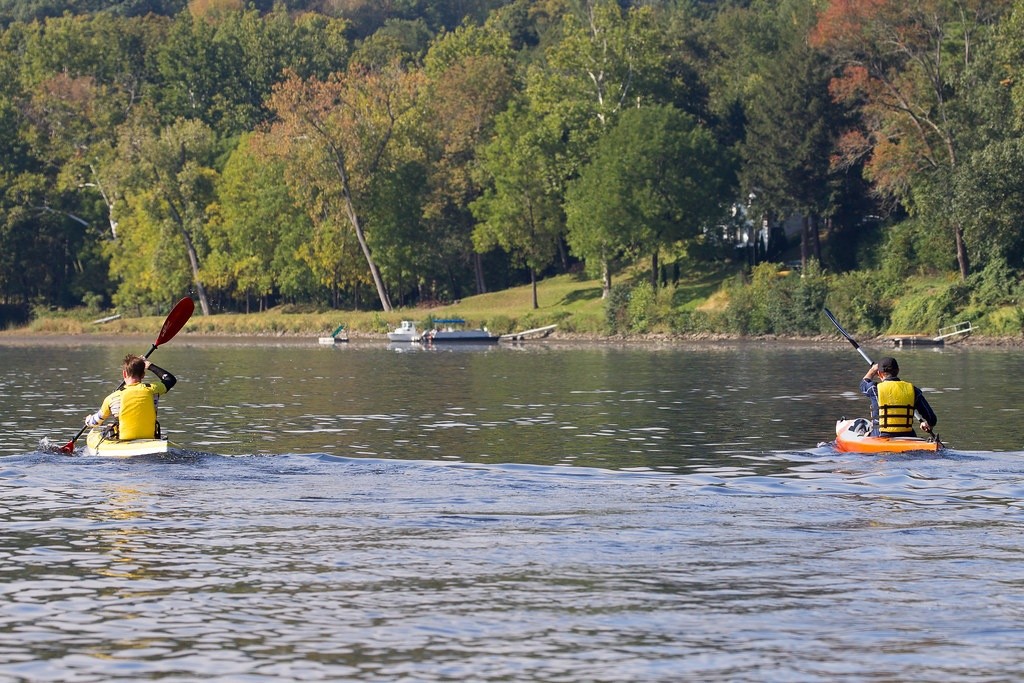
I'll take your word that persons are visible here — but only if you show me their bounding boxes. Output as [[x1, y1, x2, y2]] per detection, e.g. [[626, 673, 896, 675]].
[[859, 357, 937, 438], [85, 354, 177, 440]]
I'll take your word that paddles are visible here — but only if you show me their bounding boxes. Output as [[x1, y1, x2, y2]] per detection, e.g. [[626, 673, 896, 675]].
[[822, 307, 946, 448], [59, 296, 197, 456]]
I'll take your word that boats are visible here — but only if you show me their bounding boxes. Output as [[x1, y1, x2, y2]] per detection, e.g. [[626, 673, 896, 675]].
[[387, 320, 422, 342], [317, 325, 349, 344], [835, 415, 940, 455], [411, 317, 501, 343], [85, 424, 169, 458]]
